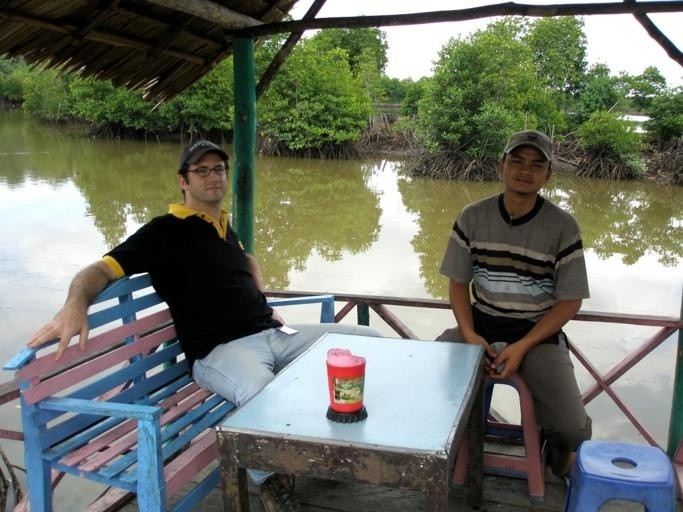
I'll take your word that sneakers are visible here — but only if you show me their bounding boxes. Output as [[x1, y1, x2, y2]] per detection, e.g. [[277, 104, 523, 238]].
[[550, 445, 571, 475], [258, 477, 302, 512]]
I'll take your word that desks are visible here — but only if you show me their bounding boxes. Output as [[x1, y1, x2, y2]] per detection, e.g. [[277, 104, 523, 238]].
[[215, 331, 485, 512]]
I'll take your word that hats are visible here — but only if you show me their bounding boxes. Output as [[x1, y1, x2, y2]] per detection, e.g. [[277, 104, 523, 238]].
[[178, 141, 229, 173], [503, 130, 553, 162]]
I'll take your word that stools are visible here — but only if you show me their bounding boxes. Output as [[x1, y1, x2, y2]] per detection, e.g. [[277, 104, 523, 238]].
[[452, 372, 547, 503], [563, 440, 676, 512]]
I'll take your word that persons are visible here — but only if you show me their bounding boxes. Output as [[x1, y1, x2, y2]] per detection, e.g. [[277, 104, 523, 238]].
[[433, 128, 593, 477], [24, 137, 385, 512]]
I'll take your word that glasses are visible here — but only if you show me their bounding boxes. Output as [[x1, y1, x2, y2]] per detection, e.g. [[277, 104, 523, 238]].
[[188, 166, 228, 177]]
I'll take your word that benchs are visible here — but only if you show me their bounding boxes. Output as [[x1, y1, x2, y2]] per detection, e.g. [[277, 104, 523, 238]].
[[3, 274, 335, 512]]
